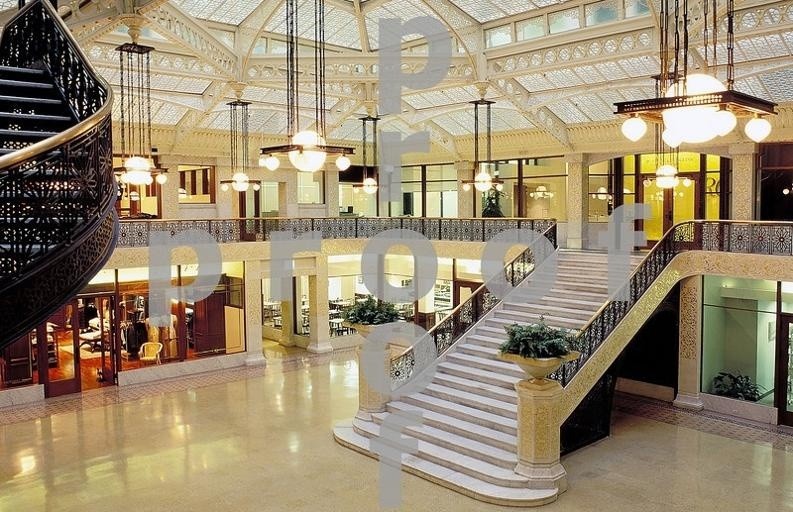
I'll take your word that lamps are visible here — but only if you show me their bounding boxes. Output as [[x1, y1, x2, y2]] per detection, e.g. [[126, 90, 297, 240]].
[[107, 0, 505, 195], [611, 4, 779, 191]]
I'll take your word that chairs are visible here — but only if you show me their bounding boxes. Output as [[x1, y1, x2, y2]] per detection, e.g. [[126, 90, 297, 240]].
[[137, 342, 163, 366]]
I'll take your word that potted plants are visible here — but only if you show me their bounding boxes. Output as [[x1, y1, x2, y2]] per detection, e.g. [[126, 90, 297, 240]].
[[493, 311, 584, 382], [340, 294, 406, 345]]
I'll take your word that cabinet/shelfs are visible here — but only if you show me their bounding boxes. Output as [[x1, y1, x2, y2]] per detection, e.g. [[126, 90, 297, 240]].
[[30, 331, 58, 368]]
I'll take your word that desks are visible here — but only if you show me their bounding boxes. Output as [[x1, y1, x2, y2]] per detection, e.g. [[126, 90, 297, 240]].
[[301, 301, 356, 336], [78, 332, 101, 352], [263, 302, 281, 331]]
